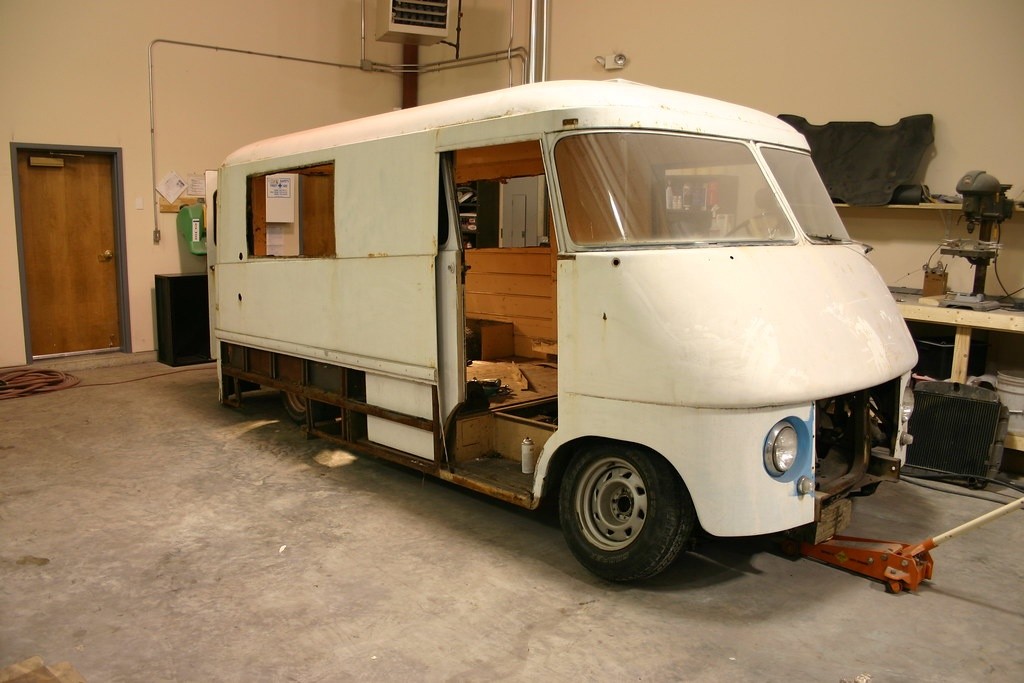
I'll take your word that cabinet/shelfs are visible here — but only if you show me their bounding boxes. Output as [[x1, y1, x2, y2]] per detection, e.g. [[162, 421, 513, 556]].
[[832, 197, 1023, 454], [156, 273, 217, 367]]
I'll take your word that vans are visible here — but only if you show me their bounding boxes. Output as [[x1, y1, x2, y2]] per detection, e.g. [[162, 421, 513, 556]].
[[207, 78, 918, 582]]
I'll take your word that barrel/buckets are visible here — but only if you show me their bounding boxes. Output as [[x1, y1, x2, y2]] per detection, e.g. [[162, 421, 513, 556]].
[[995, 369, 1024, 436]]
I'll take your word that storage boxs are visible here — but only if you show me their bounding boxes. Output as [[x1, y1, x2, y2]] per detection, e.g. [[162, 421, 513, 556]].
[[465, 319, 515, 362]]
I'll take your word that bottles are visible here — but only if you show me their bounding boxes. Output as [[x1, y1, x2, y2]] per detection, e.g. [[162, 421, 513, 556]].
[[521, 436, 535, 474], [666, 183, 691, 210]]
